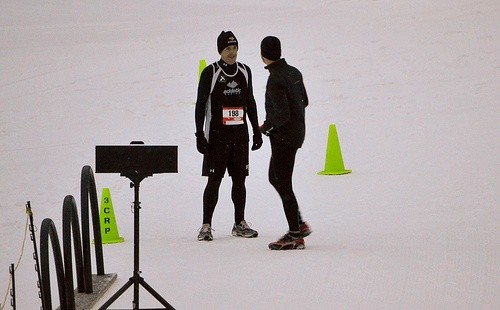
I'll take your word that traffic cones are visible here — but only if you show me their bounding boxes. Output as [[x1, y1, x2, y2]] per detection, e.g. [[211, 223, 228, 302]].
[[90, 187, 126, 244], [197, 59, 208, 80], [316, 123, 352, 176]]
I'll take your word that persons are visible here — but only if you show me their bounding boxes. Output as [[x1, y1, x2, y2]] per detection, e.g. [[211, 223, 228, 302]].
[[194, 30, 263, 241], [259, 36, 313, 250]]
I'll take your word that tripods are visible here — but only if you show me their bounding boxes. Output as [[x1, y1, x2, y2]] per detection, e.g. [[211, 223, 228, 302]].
[[93, 140, 188, 310]]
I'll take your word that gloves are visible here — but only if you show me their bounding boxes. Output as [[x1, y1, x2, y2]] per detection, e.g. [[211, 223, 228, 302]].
[[195, 131, 208, 154], [259, 121, 274, 136], [251, 133, 263, 150]]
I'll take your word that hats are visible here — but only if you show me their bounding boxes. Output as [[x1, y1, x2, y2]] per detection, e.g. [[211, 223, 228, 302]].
[[217, 30, 238, 54], [261, 36, 281, 61]]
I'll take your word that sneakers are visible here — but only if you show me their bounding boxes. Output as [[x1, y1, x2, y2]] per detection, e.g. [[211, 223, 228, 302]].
[[299, 222, 312, 237], [198, 223, 215, 241], [268, 232, 305, 250], [232, 220, 258, 238]]
